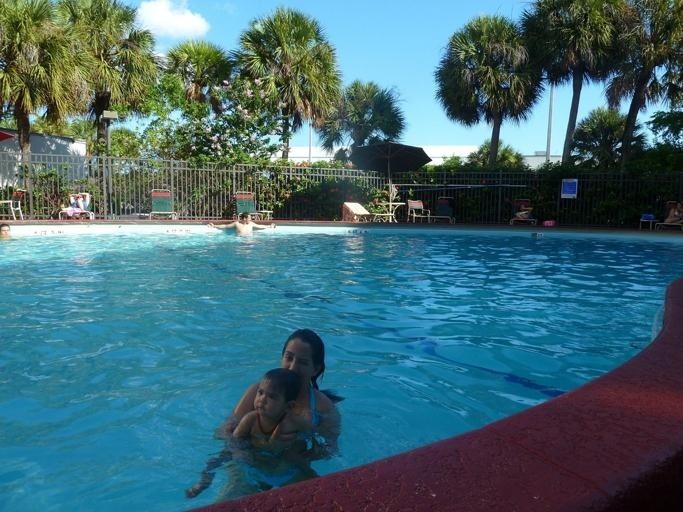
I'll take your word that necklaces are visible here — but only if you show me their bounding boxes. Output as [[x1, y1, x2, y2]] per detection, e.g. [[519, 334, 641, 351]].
[[256, 414, 285, 436]]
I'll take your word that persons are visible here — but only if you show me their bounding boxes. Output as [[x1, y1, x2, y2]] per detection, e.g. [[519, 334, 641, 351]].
[[512, 204, 533, 219], [214, 328, 339, 505], [0, 223, 10, 236], [185, 367, 310, 498], [68, 192, 90, 211], [664, 202, 682, 223], [207, 213, 275, 237]]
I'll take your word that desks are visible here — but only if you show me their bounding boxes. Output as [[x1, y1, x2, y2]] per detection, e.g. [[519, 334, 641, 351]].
[[639, 219, 660, 231]]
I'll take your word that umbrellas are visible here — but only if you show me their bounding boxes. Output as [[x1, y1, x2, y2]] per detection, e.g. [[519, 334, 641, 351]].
[[348, 140, 433, 213]]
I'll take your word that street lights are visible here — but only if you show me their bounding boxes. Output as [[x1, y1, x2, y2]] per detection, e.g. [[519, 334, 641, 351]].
[[99, 110, 118, 218]]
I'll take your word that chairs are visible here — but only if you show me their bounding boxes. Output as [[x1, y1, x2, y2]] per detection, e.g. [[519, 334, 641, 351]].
[[508, 198, 539, 227], [58, 194, 96, 222], [234, 192, 264, 220], [343, 197, 455, 224], [150, 190, 177, 220], [1, 188, 26, 221], [654, 200, 683, 234]]
